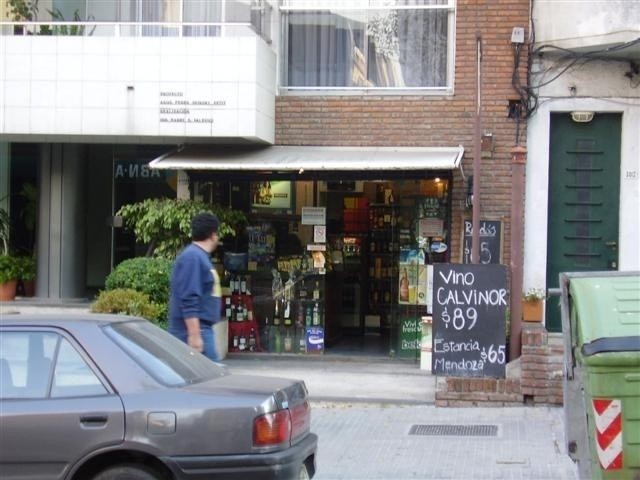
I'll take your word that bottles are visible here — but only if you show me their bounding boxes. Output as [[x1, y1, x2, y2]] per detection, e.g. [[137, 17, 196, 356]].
[[265, 275, 323, 353], [227, 273, 248, 294], [222, 297, 252, 323], [342, 244, 363, 265], [367, 207, 398, 255], [420, 195, 440, 218], [231, 327, 256, 351], [276, 256, 311, 277], [400, 268, 409, 301]]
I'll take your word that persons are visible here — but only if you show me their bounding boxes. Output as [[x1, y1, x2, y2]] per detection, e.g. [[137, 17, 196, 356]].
[[167, 213, 222, 364]]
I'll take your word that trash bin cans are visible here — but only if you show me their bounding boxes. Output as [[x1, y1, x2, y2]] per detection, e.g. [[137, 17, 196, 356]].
[[559, 271, 640, 480]]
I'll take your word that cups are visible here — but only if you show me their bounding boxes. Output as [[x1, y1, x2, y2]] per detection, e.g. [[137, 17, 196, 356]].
[[408, 285, 417, 304]]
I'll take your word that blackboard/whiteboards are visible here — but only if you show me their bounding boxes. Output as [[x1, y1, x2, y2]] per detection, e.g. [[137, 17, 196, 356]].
[[432, 263, 507, 377]]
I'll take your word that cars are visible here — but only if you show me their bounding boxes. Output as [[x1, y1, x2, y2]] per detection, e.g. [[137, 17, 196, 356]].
[[0, 313, 319, 480]]
